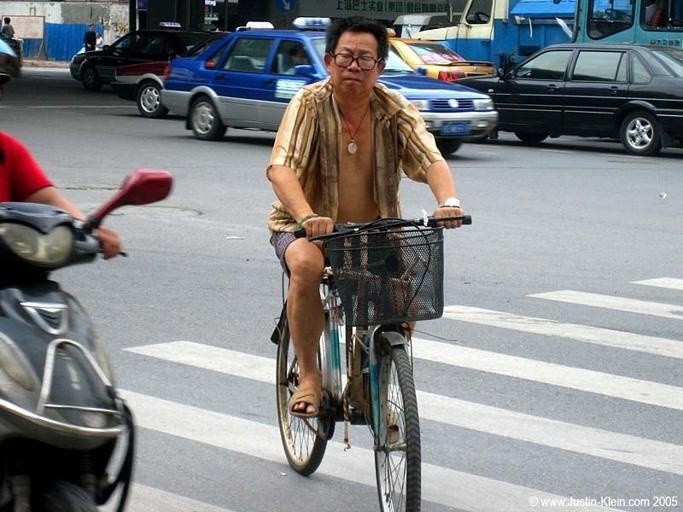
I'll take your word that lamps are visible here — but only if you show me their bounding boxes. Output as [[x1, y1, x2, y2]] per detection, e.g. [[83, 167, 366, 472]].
[[276, 54, 290, 73], [229, 56, 256, 69]]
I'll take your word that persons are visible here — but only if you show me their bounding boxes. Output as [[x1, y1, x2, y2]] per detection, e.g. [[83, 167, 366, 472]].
[[84, 24, 96, 52], [284, 42, 310, 75], [95, 32, 102, 49], [209, 19, 220, 31], [0, 17, 15, 40], [1, 129, 122, 261], [262, 18, 469, 446], [624, 0, 658, 25]]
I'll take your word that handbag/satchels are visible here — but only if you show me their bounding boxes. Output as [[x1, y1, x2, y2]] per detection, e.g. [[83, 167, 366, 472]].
[[438, 197, 461, 208], [297, 213, 319, 230]]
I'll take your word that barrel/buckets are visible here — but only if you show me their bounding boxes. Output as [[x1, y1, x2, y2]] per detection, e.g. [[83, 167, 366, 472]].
[[369, 402, 400, 445], [288, 391, 323, 418]]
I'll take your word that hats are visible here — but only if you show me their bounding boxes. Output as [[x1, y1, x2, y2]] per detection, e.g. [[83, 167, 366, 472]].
[[390, 27, 495, 82], [158, 18, 500, 153], [68, 21, 180, 93], [0, 35, 397, 106], [450, 43, 681, 154], [110, 31, 230, 117]]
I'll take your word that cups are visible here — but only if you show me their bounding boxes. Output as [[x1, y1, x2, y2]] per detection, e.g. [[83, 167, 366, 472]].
[[329, 49, 383, 72]]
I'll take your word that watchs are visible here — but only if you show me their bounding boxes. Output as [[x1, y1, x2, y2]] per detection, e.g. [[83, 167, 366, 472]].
[[1, 170, 172, 507]]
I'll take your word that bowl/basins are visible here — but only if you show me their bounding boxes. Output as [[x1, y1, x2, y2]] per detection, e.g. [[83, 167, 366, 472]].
[[338, 104, 370, 155]]
